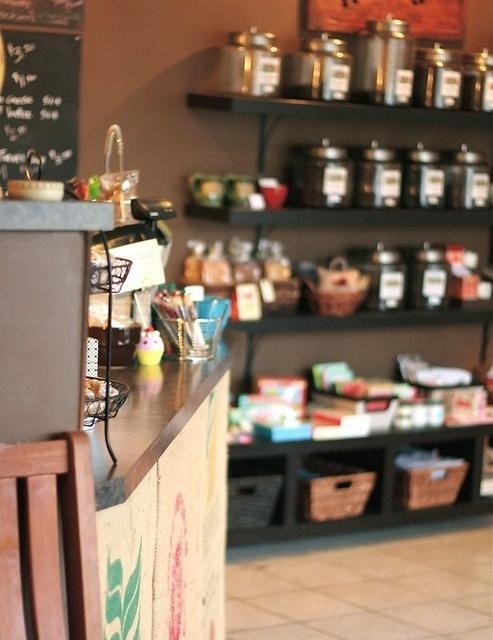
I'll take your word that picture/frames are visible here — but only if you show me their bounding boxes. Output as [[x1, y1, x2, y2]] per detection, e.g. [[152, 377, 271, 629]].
[[306, 0, 468, 42]]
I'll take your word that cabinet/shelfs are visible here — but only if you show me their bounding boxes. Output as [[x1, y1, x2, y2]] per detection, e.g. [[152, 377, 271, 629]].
[[185, 93, 493, 547]]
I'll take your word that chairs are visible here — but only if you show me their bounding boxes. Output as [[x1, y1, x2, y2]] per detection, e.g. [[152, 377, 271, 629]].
[[0, 430, 107, 640]]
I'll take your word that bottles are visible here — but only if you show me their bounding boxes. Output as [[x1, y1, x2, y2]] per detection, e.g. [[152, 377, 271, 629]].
[[298, 138, 352, 207], [401, 140, 446, 212], [442, 142, 490, 211], [413, 41, 463, 110], [219, 26, 279, 100], [287, 32, 354, 103], [182, 233, 291, 293], [404, 241, 451, 312], [355, 13, 416, 107], [463, 48, 492, 113], [354, 140, 402, 210], [357, 238, 406, 312]]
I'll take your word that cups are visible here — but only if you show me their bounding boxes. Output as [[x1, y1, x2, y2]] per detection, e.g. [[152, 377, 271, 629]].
[[258, 183, 289, 211], [186, 171, 223, 209], [222, 175, 256, 212]]
